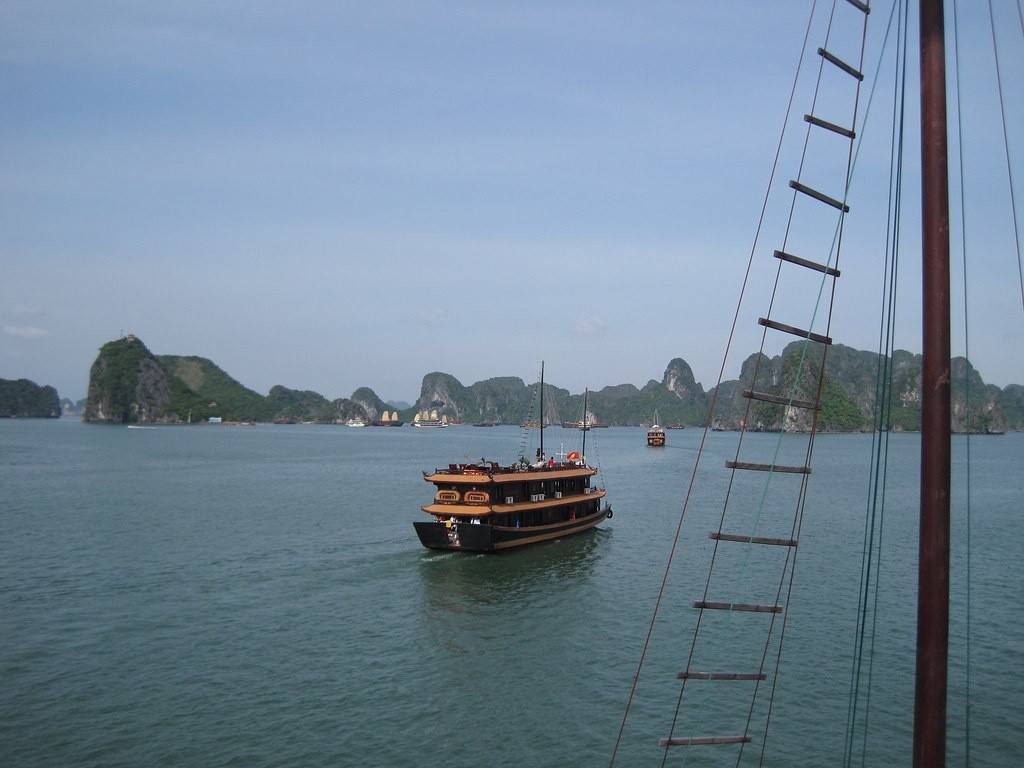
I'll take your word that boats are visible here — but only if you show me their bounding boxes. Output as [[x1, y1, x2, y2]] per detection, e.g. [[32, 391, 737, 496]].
[[413, 361, 613, 556], [578, 419, 591, 431], [348, 421, 365, 427], [647, 409, 666, 448]]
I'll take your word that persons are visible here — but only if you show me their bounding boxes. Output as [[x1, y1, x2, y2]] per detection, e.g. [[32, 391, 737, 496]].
[[548, 457, 554, 468], [517, 520, 520, 528]]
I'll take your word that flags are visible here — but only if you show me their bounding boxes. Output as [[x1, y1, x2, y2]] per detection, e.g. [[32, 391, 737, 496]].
[[567, 451, 580, 459]]
